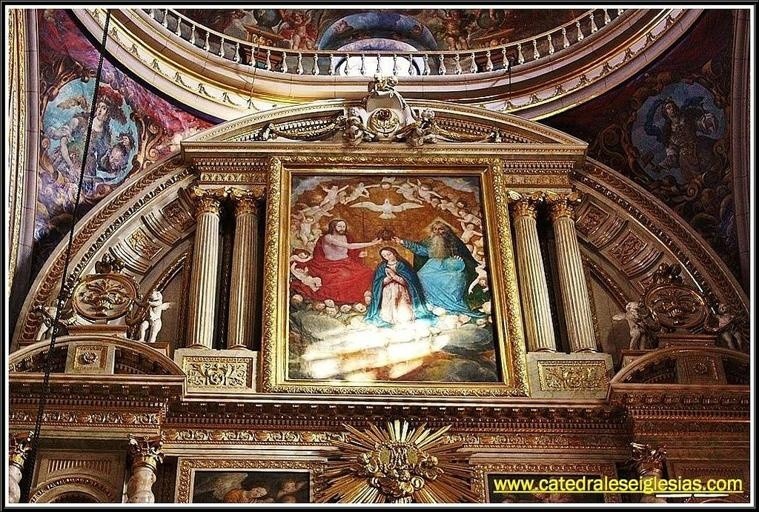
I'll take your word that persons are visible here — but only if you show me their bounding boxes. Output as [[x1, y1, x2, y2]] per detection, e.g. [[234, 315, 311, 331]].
[[50, 83, 127, 194], [279, 9, 318, 52], [220, 482, 269, 503], [289, 174, 492, 330], [499, 479, 575, 502], [135, 288, 175, 343], [116, 127, 136, 152], [611, 300, 651, 351], [653, 93, 719, 184], [437, 6, 471, 52], [278, 495, 296, 503], [274, 476, 310, 501], [32, 298, 65, 342], [712, 302, 743, 352]]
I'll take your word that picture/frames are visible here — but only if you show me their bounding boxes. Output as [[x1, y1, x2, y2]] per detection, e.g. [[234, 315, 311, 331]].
[[173, 457, 327, 503], [260, 155, 531, 398], [470, 463, 622, 504]]
